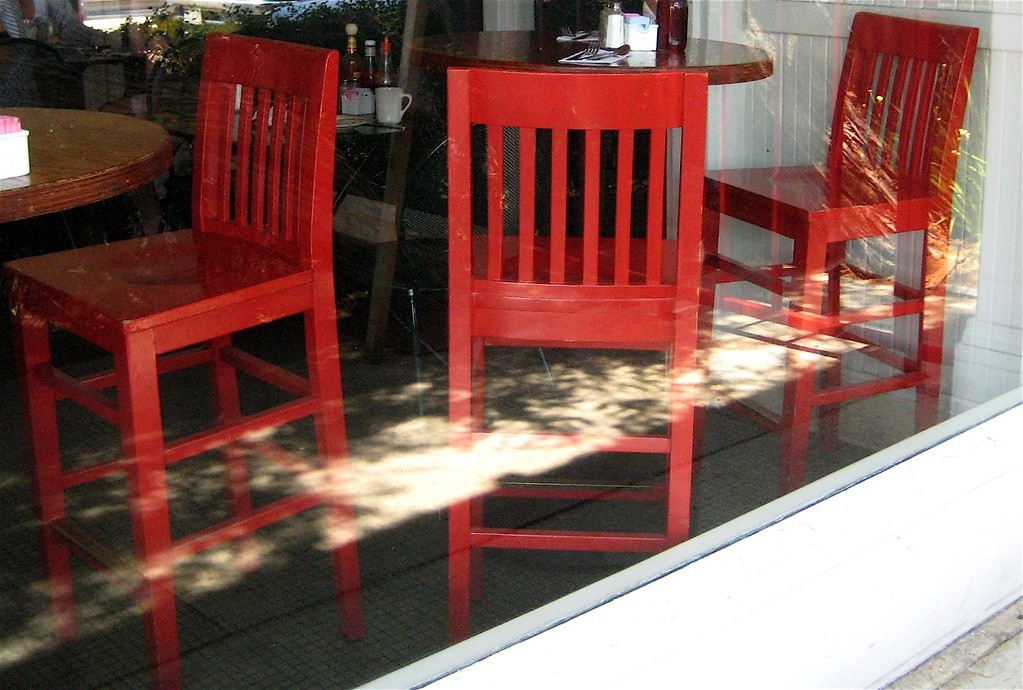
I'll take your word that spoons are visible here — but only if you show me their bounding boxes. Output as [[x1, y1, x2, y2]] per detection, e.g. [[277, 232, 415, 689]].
[[571, 29, 598, 40], [588, 45, 630, 59]]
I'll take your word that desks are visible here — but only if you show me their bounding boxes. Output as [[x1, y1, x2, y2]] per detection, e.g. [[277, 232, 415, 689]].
[[5, 33, 363, 690], [0, 106, 173, 225], [405, 30, 772, 85]]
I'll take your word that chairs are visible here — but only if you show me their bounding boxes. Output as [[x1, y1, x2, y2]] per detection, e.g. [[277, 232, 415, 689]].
[[445, 65, 708, 647], [689, 11, 978, 496]]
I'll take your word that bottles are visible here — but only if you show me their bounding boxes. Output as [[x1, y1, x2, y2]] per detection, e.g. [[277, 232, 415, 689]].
[[656, 0, 688, 52], [339, 78, 361, 110], [599, 2, 624, 50], [342, 35, 363, 81], [358, 40, 377, 94], [375, 37, 398, 87]]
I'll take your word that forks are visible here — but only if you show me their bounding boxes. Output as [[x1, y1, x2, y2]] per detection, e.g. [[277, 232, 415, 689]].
[[566, 41, 601, 60], [560, 25, 576, 37]]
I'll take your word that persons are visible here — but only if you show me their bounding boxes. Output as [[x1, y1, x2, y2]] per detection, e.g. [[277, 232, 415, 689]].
[[0, 0, 235, 236]]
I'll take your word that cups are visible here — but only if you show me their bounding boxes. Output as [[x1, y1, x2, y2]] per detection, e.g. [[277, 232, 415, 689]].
[[375, 87, 412, 124]]
